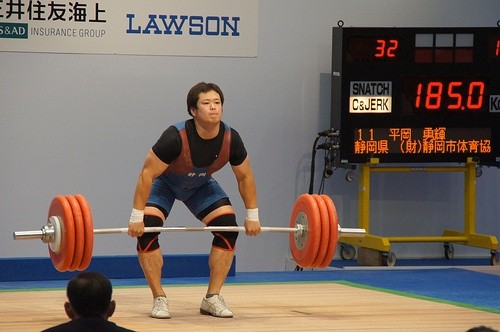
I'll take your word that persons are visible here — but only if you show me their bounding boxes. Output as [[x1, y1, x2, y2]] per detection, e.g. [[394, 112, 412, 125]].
[[41, 272, 136, 332], [128, 82, 261, 318]]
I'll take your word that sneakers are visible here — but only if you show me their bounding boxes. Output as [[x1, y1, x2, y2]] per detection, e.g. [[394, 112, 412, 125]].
[[151, 296, 171, 319], [200, 295, 233, 318]]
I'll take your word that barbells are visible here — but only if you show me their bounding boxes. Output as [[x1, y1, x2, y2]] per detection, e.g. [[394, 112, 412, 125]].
[[12, 192, 368, 274]]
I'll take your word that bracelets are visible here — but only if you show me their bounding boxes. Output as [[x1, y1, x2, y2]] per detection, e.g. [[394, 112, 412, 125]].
[[130, 208, 144, 223], [245, 208, 259, 221]]
